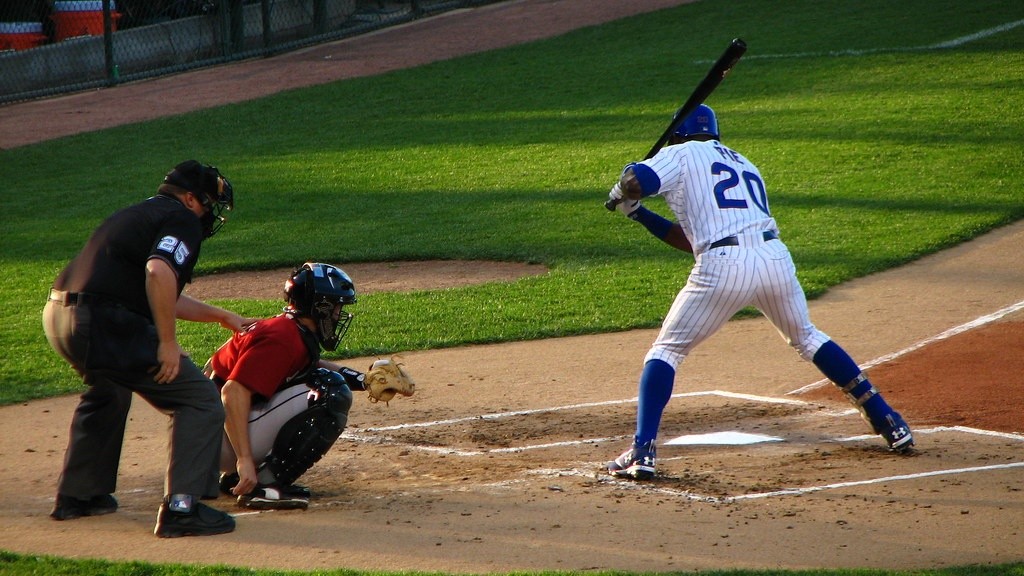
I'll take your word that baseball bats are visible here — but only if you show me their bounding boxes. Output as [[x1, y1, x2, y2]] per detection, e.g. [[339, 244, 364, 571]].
[[604, 37, 747, 213]]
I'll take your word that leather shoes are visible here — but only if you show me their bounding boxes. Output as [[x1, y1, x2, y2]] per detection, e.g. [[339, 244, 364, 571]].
[[154, 502, 236, 538], [50, 494, 117, 520]]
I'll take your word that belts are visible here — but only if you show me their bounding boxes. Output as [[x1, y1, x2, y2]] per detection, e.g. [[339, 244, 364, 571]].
[[48, 287, 77, 303], [209, 372, 225, 387], [708, 230, 778, 250]]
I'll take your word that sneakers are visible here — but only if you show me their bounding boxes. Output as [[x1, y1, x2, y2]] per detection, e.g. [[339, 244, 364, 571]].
[[219, 472, 240, 494], [883, 414, 915, 454], [605, 435, 656, 480], [237, 481, 308, 510]]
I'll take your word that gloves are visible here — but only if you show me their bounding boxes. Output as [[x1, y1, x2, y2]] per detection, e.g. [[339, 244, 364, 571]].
[[608, 181, 643, 222]]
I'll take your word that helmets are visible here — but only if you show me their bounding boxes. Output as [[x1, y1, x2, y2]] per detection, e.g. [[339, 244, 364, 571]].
[[671, 104, 719, 138], [284, 262, 357, 351]]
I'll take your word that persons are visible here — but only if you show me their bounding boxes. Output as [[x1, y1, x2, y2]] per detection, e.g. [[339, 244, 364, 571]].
[[203, 262, 415, 512], [607, 104, 915, 478], [43, 159, 259, 539]]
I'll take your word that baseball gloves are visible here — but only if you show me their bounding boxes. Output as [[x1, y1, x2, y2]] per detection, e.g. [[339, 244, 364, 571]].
[[365, 359, 416, 402]]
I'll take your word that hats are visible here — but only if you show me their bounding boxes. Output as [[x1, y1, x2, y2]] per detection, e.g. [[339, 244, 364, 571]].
[[164, 160, 232, 202]]
